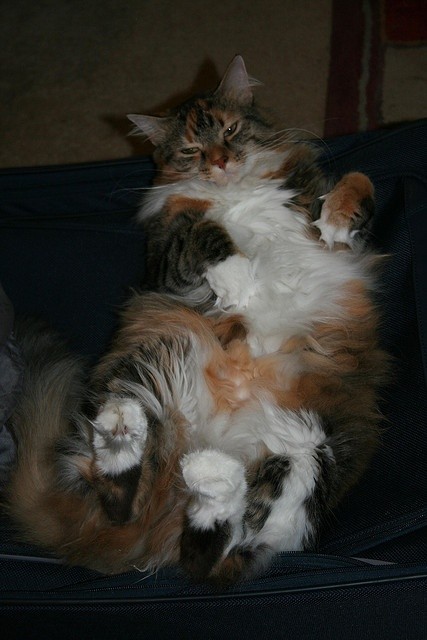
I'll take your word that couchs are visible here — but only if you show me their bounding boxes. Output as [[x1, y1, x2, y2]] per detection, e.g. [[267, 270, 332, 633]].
[[1, 118, 426, 638]]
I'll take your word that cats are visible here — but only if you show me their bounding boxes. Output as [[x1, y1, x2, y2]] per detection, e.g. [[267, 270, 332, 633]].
[[0, 54, 396, 584]]
